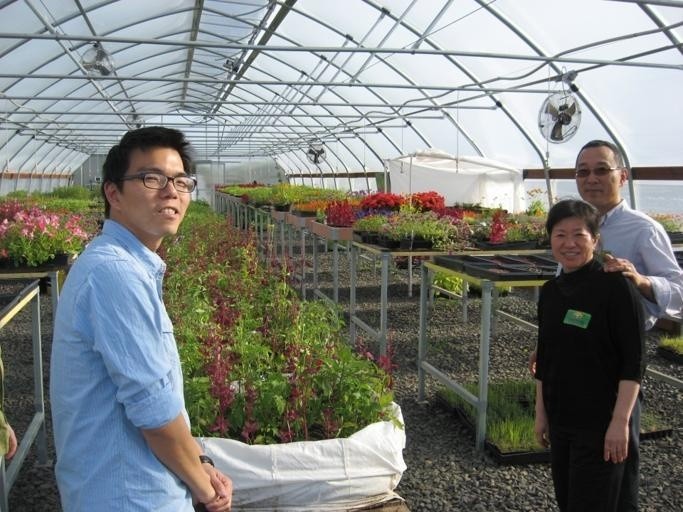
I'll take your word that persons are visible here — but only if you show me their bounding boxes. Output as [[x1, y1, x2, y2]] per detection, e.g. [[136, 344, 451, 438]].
[[529, 140, 683, 376], [534, 199, 647, 511], [49, 127, 232, 512]]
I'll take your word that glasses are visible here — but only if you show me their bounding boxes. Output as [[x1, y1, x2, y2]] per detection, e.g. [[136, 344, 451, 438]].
[[119, 172, 196, 193], [574, 167, 621, 177]]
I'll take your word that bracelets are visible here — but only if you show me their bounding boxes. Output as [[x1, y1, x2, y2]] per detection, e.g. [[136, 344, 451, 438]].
[[199, 456, 214, 468]]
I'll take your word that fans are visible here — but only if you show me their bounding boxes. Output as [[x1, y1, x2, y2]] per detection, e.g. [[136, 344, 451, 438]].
[[126, 107, 146, 129], [79, 43, 114, 81], [538, 70, 581, 144], [306, 137, 327, 165]]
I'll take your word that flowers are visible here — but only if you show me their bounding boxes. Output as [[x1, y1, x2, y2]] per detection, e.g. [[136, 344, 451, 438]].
[[0, 184, 405, 445], [215, 180, 682, 253]]
